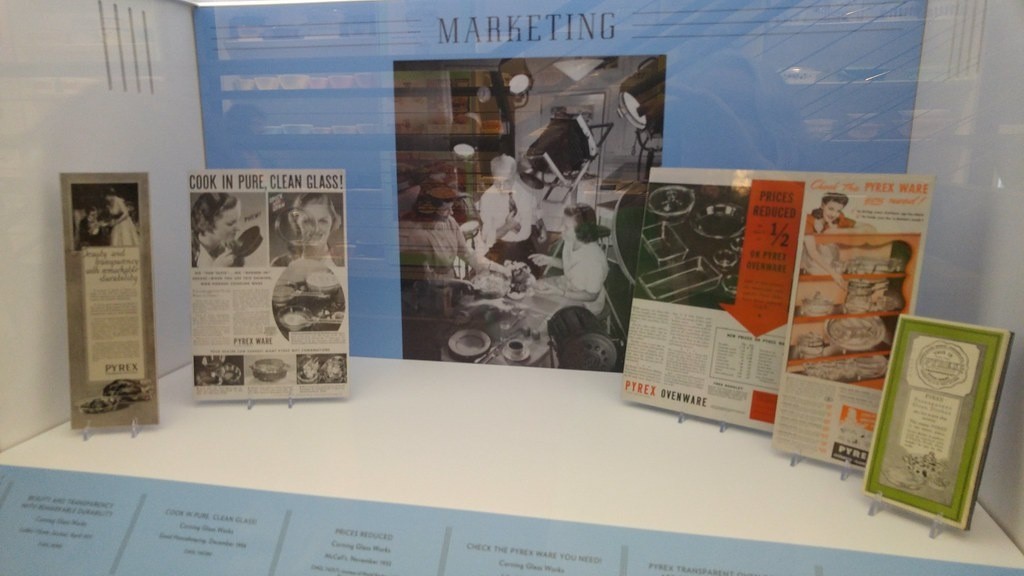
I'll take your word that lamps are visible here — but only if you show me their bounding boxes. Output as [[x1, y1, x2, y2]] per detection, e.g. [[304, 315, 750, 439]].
[[500, 58, 532, 96], [619, 57, 664, 131]]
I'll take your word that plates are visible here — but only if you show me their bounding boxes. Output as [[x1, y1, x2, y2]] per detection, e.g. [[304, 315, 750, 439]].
[[446, 329, 492, 357], [501, 344, 531, 360], [790, 254, 888, 381]]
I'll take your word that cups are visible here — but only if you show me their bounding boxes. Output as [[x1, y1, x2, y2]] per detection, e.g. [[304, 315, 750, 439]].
[[507, 340, 524, 355], [720, 273, 739, 303], [730, 236, 745, 258], [711, 248, 737, 272]]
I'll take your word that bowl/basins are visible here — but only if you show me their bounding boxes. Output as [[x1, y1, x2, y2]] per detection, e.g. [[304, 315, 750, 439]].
[[647, 184, 696, 225], [638, 255, 723, 302], [689, 201, 750, 245], [641, 217, 689, 268]]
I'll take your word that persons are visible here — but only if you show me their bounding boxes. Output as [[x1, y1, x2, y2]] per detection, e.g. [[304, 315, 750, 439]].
[[77, 204, 113, 246], [404, 182, 515, 296], [476, 154, 548, 279], [188, 192, 266, 268], [525, 200, 612, 317], [97, 186, 139, 246], [269, 190, 345, 268], [401, 250, 454, 360]]
